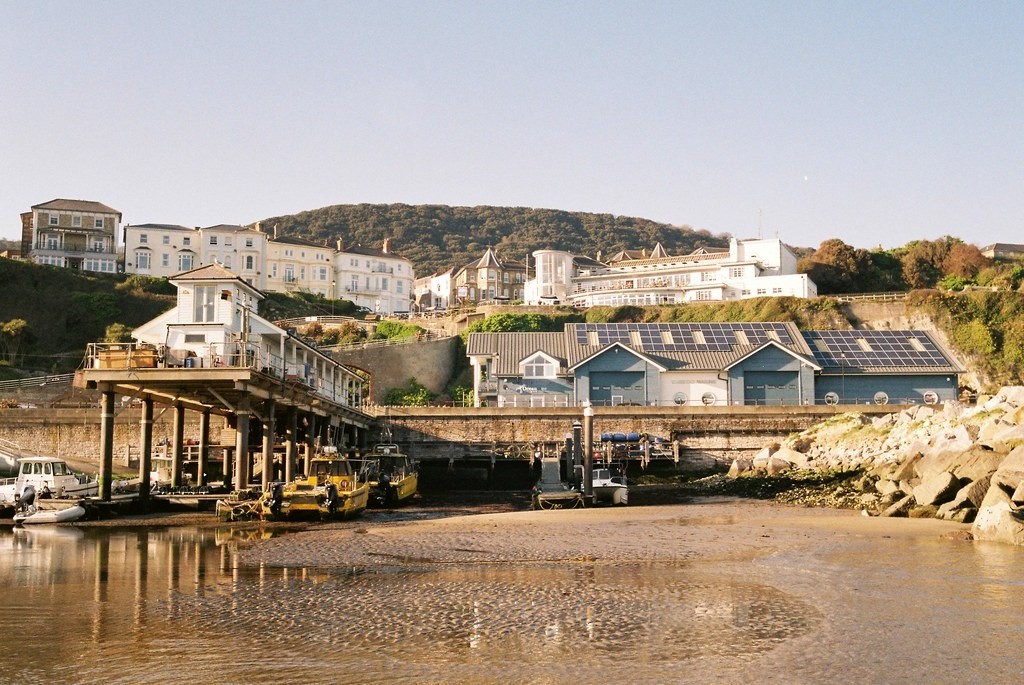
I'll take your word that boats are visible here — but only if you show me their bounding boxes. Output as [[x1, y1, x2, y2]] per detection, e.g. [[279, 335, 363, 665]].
[[355, 419, 422, 504], [565, 463, 629, 506], [261, 437, 373, 517], [0, 454, 99, 523]]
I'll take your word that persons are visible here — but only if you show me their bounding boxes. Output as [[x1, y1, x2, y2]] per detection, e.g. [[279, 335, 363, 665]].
[[55, 486, 69, 499], [39, 486, 52, 499]]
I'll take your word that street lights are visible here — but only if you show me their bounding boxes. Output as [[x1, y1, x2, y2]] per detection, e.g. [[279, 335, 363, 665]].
[[495, 352, 500, 407], [333, 280, 336, 317], [841, 350, 846, 404]]
